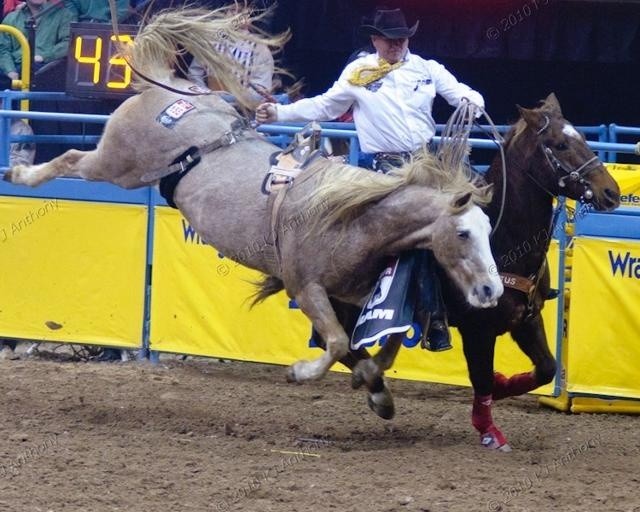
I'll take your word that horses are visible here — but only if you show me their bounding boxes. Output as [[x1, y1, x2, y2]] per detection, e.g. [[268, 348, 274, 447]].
[[1, 1, 505, 421], [239, 91, 621, 453]]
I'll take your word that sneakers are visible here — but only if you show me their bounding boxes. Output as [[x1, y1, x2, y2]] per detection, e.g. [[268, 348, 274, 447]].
[[429, 320, 452, 352]]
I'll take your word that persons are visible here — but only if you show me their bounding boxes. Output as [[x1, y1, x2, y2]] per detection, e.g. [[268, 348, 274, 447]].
[[0, 1, 145, 166], [184, 1, 276, 121], [251, 8, 486, 352]]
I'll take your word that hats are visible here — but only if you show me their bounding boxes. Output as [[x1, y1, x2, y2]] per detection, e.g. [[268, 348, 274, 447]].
[[359, 8, 420, 40]]
